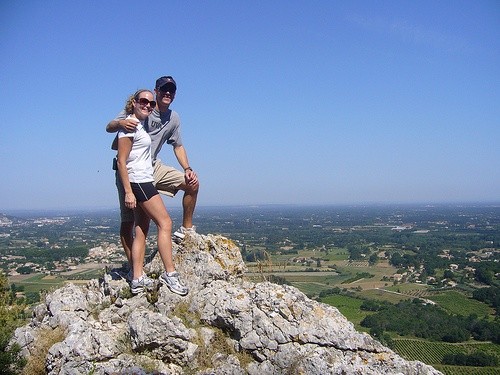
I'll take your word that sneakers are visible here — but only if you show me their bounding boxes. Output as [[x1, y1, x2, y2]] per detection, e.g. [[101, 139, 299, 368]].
[[159, 270, 188, 296], [126, 269, 160, 288], [173, 224, 204, 246], [131, 276, 162, 295]]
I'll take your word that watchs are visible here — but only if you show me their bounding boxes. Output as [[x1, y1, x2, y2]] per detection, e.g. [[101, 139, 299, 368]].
[[182, 165, 194, 172]]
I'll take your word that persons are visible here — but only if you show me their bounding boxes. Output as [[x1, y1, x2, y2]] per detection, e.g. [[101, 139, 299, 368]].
[[104, 74, 200, 274], [114, 89, 191, 297]]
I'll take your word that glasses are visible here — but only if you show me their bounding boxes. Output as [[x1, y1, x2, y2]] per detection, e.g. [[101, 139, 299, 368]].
[[136, 98, 156, 108], [156, 86, 177, 94]]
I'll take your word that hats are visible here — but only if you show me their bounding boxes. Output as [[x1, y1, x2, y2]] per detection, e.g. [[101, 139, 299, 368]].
[[156, 75, 177, 91]]
